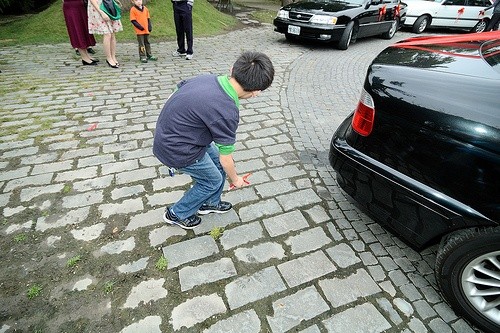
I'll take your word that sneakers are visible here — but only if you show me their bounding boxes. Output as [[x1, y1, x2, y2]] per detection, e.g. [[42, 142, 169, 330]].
[[148, 56, 158, 60], [140, 57, 148, 63], [173, 51, 187, 56], [163, 207, 202, 229], [185, 54, 193, 60], [197, 201, 231, 214]]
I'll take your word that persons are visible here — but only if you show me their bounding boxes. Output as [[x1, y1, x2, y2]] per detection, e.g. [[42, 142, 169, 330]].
[[130, 0, 158, 63], [62, 0, 100, 65], [171, 0, 196, 60], [87, 0, 125, 68], [153, 52, 276, 229]]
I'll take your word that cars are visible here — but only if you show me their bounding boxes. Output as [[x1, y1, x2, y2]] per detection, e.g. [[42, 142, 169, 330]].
[[403, 0, 495, 33], [273, 0, 408, 50], [328, 31, 500, 333]]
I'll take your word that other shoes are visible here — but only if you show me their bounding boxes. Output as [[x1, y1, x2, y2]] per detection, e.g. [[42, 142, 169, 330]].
[[87, 48, 96, 54], [75, 50, 80, 55]]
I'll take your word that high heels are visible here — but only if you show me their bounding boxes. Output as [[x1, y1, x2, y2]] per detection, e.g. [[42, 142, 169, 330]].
[[82, 58, 99, 65], [106, 59, 119, 68]]
